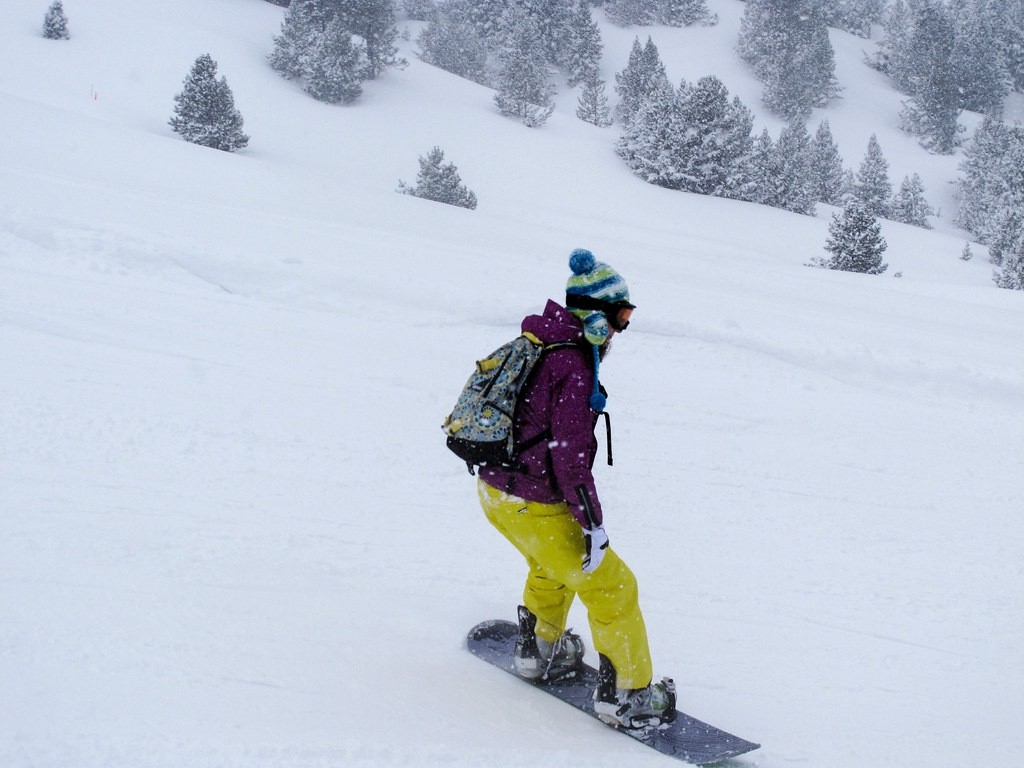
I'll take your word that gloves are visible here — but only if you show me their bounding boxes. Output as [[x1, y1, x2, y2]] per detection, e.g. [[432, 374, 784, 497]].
[[581, 527, 609, 572]]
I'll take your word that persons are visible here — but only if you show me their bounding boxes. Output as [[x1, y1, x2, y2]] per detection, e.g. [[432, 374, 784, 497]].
[[440, 246, 674, 733]]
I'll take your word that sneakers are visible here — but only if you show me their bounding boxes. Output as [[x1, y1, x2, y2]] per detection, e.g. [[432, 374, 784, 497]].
[[513, 636, 585, 680], [595, 684, 677, 728]]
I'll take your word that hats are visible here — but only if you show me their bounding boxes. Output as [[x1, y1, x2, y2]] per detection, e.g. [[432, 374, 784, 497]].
[[564, 248, 630, 345]]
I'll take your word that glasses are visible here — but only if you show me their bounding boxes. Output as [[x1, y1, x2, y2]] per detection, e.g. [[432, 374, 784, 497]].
[[566, 294, 635, 333]]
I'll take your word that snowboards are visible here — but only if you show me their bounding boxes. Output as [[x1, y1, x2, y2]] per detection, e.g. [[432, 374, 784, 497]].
[[468, 619, 761, 765]]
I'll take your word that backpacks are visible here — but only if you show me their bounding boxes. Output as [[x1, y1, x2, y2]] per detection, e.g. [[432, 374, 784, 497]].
[[442, 332, 593, 475]]
